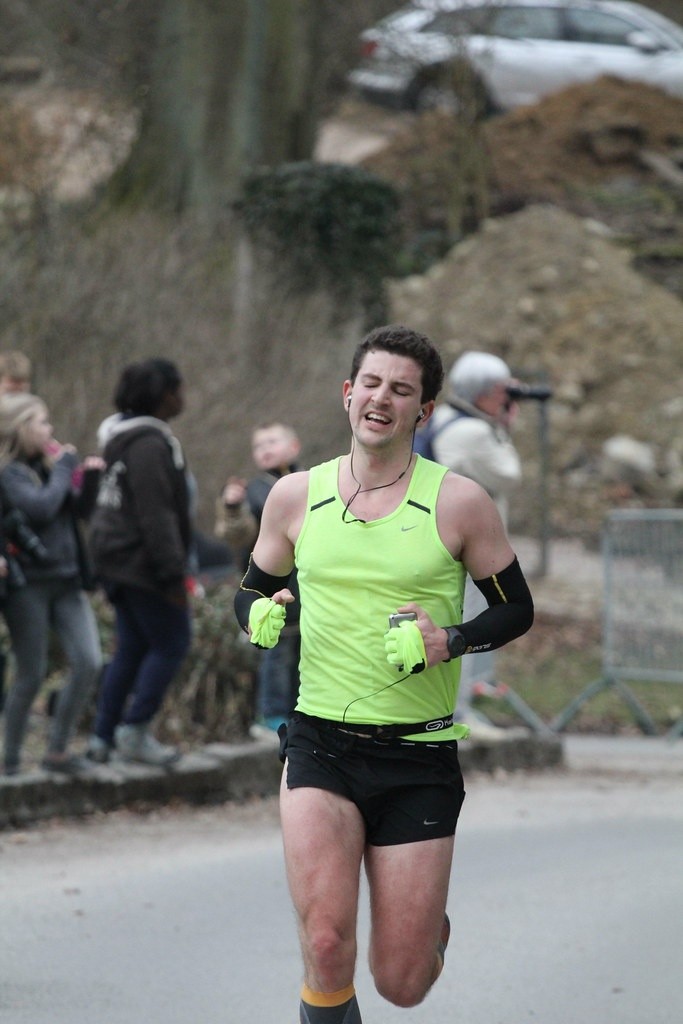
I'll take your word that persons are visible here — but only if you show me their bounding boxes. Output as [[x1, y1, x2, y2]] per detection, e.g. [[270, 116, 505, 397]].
[[89, 356, 193, 764], [213, 417, 305, 746], [428, 351, 525, 730], [0, 351, 104, 779], [231, 324, 535, 1024]]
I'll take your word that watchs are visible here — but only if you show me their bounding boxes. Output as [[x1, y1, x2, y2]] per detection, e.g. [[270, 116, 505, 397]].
[[440, 624, 465, 663]]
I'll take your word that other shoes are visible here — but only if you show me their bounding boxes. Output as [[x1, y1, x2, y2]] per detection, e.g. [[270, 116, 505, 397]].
[[118, 737, 179, 764], [0, 766, 35, 788], [456, 715, 507, 748], [37, 758, 109, 783]]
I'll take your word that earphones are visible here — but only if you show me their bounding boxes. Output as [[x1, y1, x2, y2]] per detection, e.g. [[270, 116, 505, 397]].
[[415, 409, 425, 424], [347, 395, 352, 407]]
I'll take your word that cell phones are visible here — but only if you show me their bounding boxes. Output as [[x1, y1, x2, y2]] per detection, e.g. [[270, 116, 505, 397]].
[[389, 613, 417, 630]]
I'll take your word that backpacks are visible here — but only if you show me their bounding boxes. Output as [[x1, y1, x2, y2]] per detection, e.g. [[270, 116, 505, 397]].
[[412, 411, 468, 462]]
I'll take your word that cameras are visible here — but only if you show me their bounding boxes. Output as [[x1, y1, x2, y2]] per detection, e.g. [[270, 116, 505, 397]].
[[505, 382, 553, 412]]
